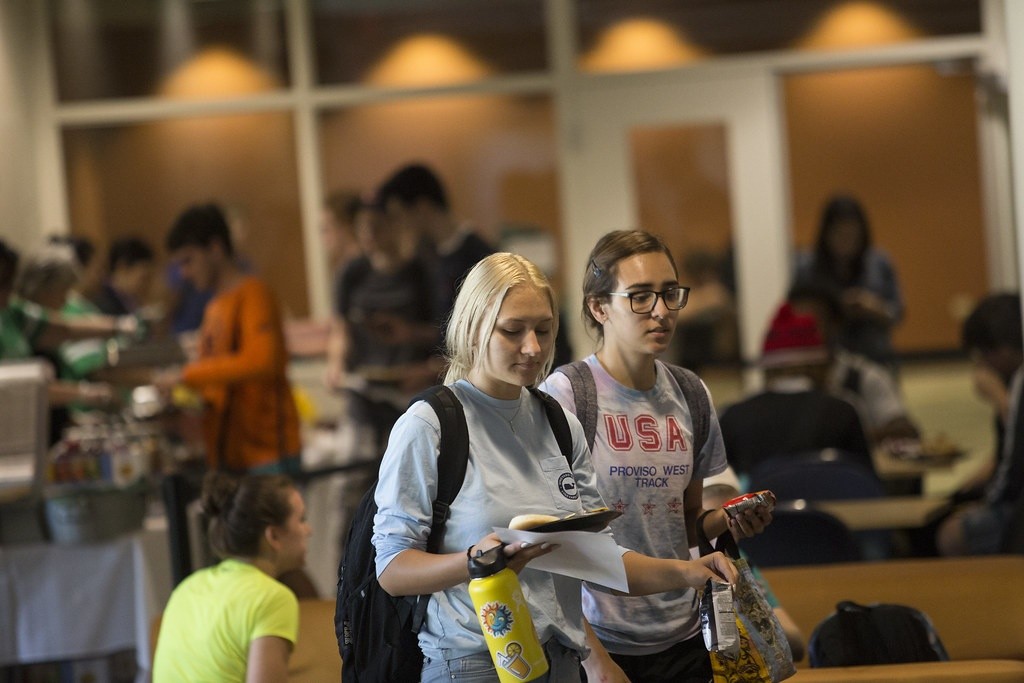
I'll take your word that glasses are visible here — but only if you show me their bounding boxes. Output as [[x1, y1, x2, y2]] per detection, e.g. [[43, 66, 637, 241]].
[[603, 286, 690, 314]]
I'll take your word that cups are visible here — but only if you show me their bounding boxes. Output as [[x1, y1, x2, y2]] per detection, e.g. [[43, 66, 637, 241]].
[[44, 494, 91, 546]]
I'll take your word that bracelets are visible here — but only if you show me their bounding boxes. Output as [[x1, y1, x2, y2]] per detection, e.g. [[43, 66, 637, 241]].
[[112, 317, 117, 333], [467, 545, 476, 559], [80, 382, 89, 400]]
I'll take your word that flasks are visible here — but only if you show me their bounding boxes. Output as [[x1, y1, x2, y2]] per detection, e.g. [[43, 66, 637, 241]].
[[468, 542, 550, 683]]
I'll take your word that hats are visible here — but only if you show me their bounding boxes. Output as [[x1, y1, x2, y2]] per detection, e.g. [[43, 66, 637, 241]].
[[751, 302, 828, 368]]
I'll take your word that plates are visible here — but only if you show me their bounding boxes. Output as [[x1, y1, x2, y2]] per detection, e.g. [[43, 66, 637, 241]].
[[522, 506, 626, 533]]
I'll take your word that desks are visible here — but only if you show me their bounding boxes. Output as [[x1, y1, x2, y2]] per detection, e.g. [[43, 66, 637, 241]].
[[760, 557, 1024, 668], [0, 504, 203, 683]]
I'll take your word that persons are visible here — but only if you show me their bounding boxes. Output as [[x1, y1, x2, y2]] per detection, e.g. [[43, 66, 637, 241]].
[[815, 192, 909, 387], [539, 230, 772, 683], [924, 290, 1023, 557], [0, 231, 156, 439], [153, 473, 311, 682], [151, 202, 301, 472], [370, 251, 738, 683], [326, 162, 497, 441], [702, 269, 909, 560]]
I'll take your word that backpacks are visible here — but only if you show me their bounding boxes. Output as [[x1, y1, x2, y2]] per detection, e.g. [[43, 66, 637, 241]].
[[335, 387, 571, 683]]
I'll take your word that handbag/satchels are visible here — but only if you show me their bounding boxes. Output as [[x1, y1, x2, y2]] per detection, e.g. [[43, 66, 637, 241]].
[[694, 509, 796, 683]]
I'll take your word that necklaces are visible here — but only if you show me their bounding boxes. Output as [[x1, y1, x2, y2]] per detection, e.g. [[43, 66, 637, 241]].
[[463, 374, 522, 435]]
